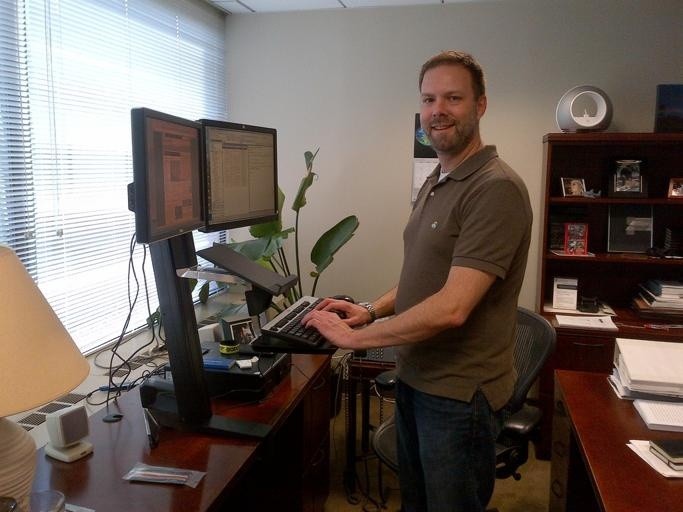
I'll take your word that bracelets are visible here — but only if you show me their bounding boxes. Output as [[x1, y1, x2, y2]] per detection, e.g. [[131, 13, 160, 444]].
[[356, 300, 377, 326]]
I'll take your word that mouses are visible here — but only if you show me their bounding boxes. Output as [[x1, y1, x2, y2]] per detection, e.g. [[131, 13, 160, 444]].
[[332, 295, 354, 304]]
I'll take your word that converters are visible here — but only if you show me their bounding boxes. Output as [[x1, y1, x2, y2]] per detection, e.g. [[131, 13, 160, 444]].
[[99, 383, 136, 391]]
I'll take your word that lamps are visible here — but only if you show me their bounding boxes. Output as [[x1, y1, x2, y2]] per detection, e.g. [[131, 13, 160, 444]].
[[0, 244, 93, 512]]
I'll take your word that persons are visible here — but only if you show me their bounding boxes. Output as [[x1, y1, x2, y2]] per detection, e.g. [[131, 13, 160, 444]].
[[300, 49, 534, 510]]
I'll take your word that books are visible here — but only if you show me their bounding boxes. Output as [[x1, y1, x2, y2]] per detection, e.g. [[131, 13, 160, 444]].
[[606, 203, 655, 253], [635, 278, 682, 313], [606, 338, 683, 472], [556, 315, 618, 332]]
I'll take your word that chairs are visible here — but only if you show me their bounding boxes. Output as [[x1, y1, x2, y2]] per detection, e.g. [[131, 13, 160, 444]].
[[368, 307, 555, 512]]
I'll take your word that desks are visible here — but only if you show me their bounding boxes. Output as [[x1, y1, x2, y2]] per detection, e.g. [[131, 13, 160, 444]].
[[548, 369, 682, 511]]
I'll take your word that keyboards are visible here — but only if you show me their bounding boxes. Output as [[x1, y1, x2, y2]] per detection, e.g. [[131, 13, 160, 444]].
[[260, 295, 345, 347]]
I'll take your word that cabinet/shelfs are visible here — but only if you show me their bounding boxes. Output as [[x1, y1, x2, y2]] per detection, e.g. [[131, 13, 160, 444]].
[[533, 133, 682, 461]]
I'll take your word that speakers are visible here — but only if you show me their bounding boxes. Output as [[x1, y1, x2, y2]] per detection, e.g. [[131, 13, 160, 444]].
[[45, 406, 94, 463]]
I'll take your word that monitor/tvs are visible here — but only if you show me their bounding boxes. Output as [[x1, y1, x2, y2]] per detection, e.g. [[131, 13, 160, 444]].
[[130, 107, 207, 244], [195, 119, 279, 233]]
